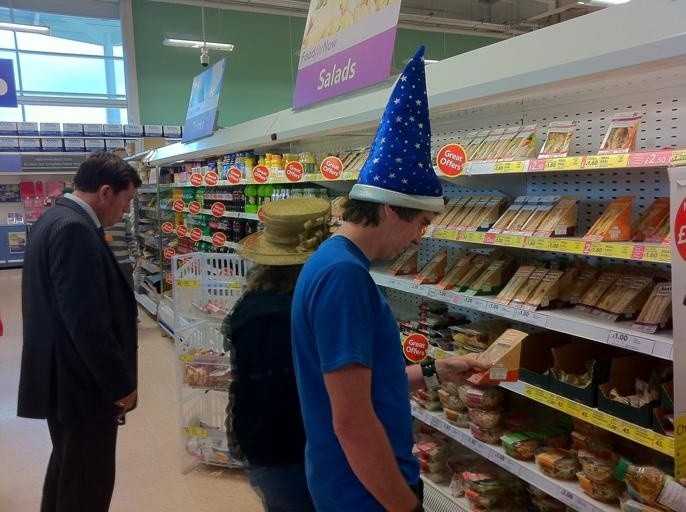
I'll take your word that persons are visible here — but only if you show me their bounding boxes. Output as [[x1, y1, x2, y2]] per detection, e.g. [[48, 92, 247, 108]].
[[287, 198, 504, 511], [14, 150, 144, 512], [220, 195, 334, 510]]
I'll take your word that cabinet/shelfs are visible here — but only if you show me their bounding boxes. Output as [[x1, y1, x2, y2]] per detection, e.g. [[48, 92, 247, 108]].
[[1, 153, 129, 269], [135, 0, 684, 510]]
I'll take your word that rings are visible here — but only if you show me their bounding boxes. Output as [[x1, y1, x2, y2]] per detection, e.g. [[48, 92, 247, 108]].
[[118, 401, 127, 406]]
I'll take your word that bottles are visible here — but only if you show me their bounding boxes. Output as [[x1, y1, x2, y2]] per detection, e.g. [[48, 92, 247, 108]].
[[173, 152, 328, 275], [613, 459, 686, 511]]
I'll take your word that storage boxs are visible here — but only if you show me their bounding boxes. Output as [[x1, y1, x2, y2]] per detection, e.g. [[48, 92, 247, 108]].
[[0, 121, 185, 152]]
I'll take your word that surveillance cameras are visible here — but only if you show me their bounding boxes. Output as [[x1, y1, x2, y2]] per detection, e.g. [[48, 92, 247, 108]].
[[200, 54, 209, 66]]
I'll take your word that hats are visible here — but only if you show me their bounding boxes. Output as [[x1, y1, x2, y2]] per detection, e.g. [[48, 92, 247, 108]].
[[346, 45, 446, 215], [234, 196, 335, 267]]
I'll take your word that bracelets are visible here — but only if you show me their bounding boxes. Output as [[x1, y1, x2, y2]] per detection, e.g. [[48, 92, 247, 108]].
[[421, 358, 442, 391]]
